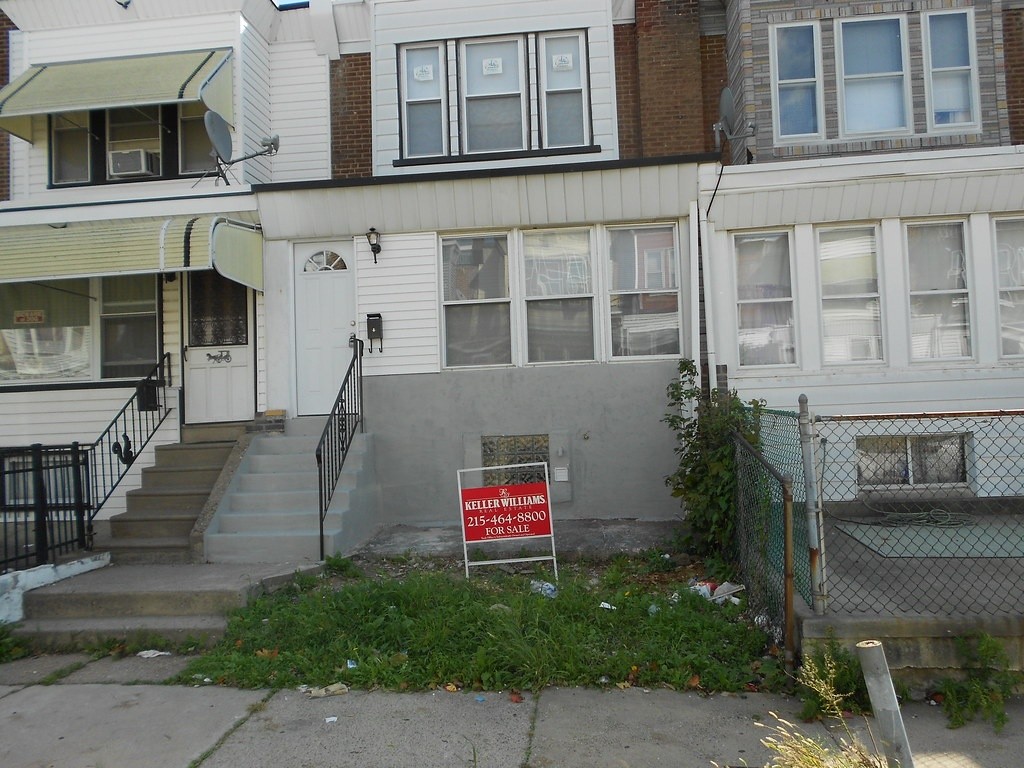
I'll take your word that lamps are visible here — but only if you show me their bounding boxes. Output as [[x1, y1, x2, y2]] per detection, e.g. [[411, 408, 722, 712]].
[[365, 228, 382, 264]]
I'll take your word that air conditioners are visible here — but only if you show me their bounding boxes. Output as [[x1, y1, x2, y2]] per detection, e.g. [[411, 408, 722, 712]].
[[108, 149, 148, 176]]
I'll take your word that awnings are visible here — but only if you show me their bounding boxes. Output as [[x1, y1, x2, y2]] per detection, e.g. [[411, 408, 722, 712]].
[[0, 51, 233, 144], [0, 216, 263, 294]]
